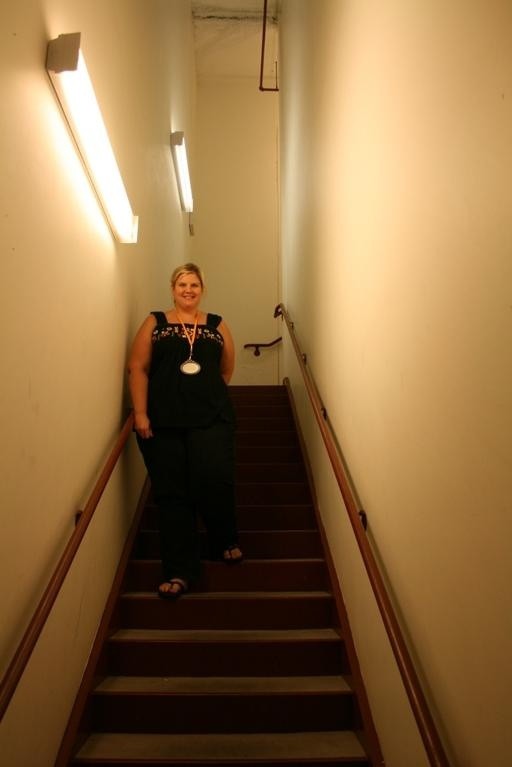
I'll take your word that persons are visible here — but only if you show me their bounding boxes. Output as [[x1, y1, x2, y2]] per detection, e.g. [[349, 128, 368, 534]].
[[126, 262, 247, 603]]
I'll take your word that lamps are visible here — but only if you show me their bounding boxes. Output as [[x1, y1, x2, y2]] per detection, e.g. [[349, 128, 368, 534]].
[[45, 30, 140, 245], [170, 130, 195, 213]]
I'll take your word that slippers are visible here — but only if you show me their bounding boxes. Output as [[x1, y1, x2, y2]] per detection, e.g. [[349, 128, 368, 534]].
[[222, 544, 243, 565], [159, 579, 193, 598]]
